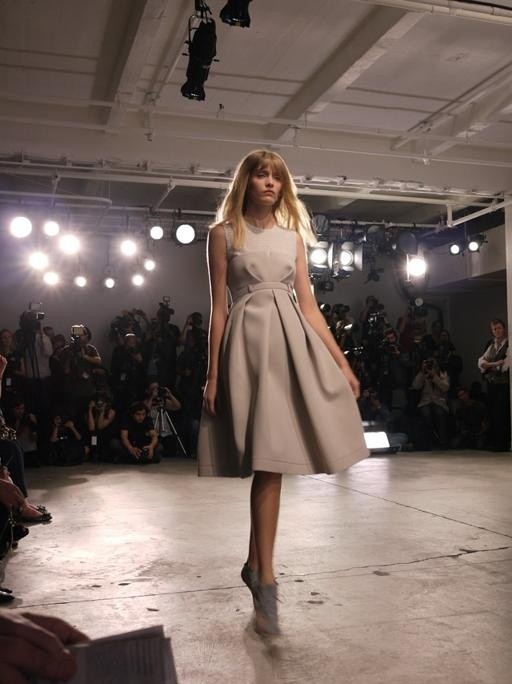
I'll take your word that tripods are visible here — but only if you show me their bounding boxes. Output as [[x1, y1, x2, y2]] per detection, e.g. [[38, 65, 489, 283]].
[[153, 397, 188, 458]]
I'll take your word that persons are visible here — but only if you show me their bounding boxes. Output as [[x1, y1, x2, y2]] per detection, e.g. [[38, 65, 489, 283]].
[[194, 148, 372, 636], [0, 607, 92, 683], [0, 467, 53, 606], [0, 295, 510, 467]]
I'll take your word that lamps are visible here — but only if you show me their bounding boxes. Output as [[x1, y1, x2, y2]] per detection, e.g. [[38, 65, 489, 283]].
[[448, 234, 489, 257], [362, 430, 394, 457], [308, 232, 364, 281], [169, 223, 198, 248], [179, 0, 252, 102]]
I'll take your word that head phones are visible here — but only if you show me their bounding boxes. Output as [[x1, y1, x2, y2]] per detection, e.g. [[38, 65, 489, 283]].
[[19, 311, 29, 329]]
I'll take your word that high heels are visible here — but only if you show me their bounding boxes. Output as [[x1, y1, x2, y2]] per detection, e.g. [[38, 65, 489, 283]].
[[240, 561, 286, 640]]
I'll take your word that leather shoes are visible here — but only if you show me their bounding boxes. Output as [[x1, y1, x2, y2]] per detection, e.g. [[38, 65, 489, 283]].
[[0, 586, 13, 594], [16, 511, 52, 522], [0, 591, 15, 604], [35, 503, 46, 511]]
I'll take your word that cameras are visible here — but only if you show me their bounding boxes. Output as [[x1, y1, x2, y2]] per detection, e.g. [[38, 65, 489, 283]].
[[158, 386, 166, 397], [368, 389, 380, 399], [61, 416, 69, 424], [189, 318, 203, 327], [95, 401, 103, 408], [421, 359, 434, 374]]
